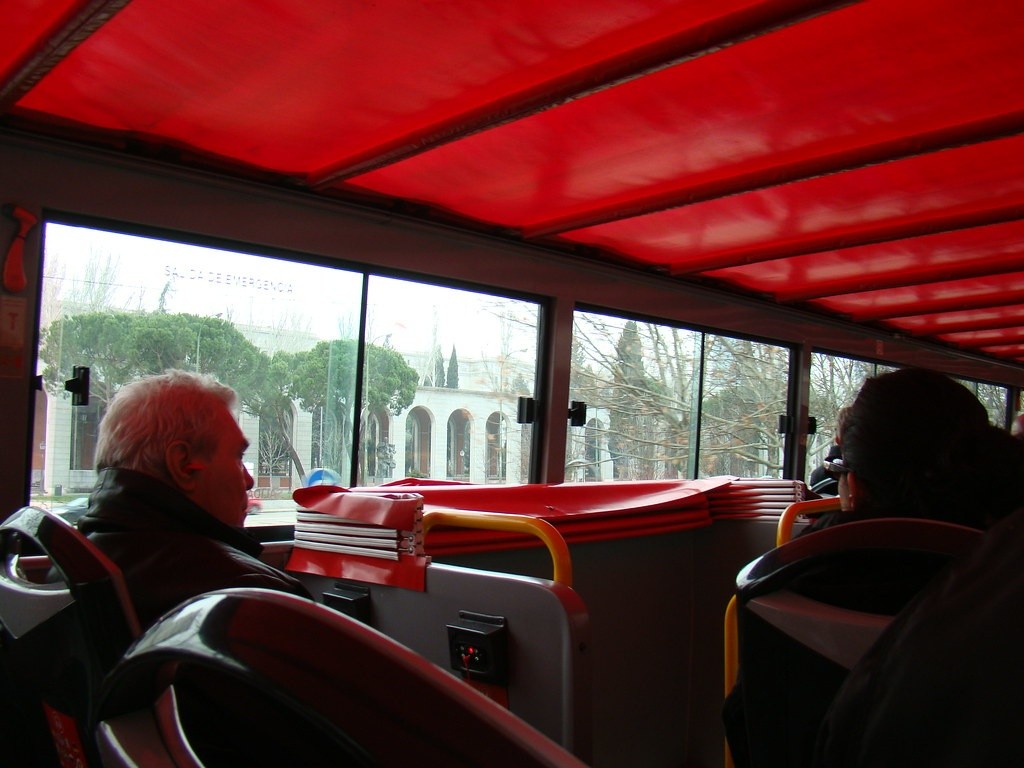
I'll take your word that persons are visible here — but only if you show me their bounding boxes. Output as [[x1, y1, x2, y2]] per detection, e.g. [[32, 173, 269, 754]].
[[77, 368, 367, 766], [721, 366, 1024, 767]]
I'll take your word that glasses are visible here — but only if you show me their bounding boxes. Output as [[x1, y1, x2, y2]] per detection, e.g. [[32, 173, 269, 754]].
[[823, 454, 855, 481]]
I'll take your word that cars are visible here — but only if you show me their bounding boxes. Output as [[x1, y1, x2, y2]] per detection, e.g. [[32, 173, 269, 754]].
[[247, 495, 262, 516]]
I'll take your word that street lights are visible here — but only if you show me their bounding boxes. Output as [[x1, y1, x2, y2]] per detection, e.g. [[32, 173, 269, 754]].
[[594, 360, 625, 481], [498, 347, 528, 485], [196, 311, 224, 374], [363, 333, 393, 486]]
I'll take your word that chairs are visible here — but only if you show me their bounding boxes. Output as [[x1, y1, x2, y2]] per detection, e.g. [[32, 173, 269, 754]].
[[0, 497, 1024, 768]]
